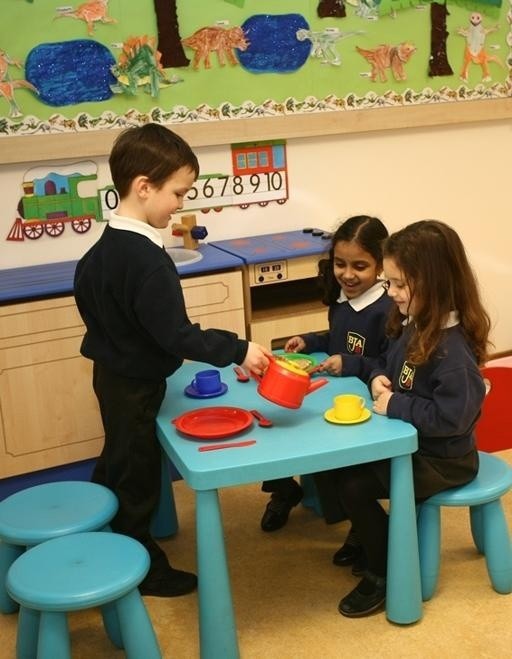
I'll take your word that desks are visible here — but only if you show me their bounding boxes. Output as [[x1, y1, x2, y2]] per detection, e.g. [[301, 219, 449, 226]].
[[141, 341, 422, 659]]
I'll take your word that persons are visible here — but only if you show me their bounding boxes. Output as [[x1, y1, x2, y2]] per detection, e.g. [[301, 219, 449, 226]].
[[260, 216, 395, 565], [73, 123, 274, 596], [337, 220, 491, 617]]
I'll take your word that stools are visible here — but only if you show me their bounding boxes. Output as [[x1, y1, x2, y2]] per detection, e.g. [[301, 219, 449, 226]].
[[297, 472, 379, 521], [6, 531, 163, 659], [415, 448, 510, 601], [1, 481, 120, 614]]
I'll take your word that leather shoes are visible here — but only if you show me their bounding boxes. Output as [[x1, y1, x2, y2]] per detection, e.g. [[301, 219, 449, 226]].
[[259, 477, 304, 532], [135, 566, 199, 598], [332, 516, 390, 575], [335, 575, 386, 619]]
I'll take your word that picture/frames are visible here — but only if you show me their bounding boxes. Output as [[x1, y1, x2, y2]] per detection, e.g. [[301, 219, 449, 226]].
[[1, 1, 512, 163]]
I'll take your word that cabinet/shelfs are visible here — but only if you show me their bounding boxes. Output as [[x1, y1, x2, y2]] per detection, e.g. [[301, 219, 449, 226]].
[[1, 242, 246, 480], [210, 228, 333, 350]]
